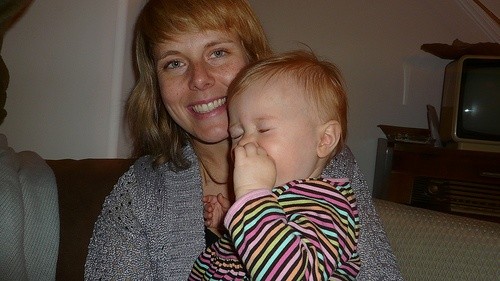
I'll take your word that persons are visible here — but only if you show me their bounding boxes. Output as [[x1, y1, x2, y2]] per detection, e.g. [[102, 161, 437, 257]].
[[186, 47, 362, 281], [82, 0, 404, 281]]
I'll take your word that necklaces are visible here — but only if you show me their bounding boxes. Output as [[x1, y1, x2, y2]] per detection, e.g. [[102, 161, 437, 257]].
[[197, 157, 228, 186]]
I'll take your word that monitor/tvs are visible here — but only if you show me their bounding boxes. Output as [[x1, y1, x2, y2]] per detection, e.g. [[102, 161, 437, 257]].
[[451, 56, 499, 152]]
